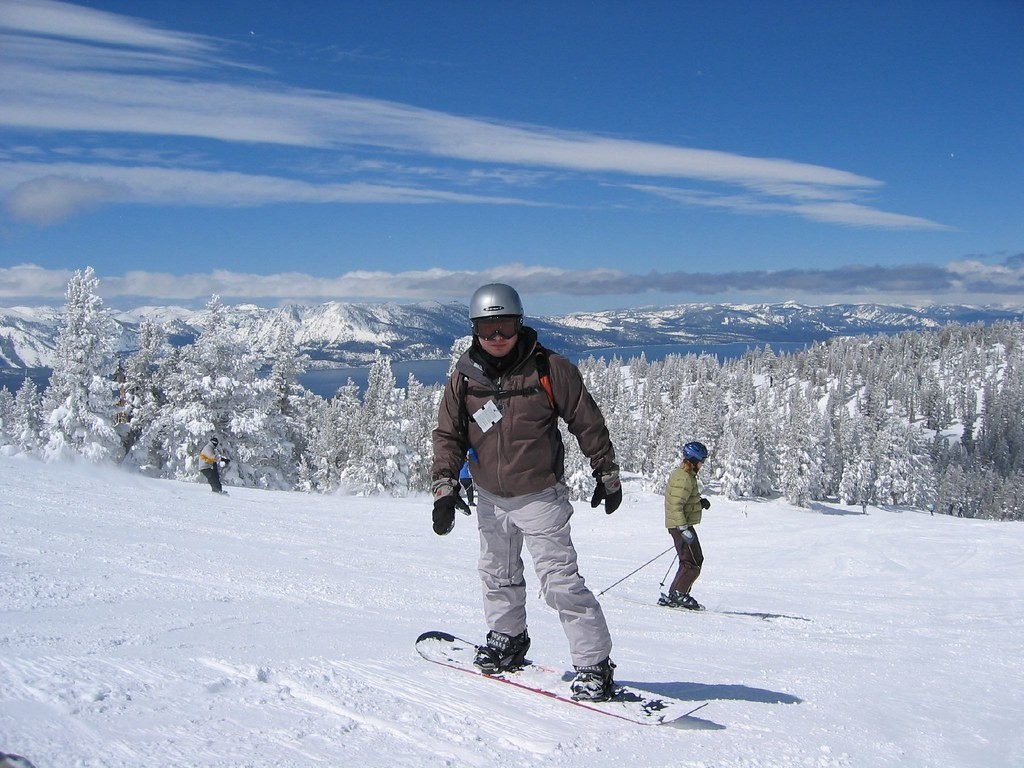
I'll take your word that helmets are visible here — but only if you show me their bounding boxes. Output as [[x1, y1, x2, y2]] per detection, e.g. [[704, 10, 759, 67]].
[[210, 438, 218, 447], [682, 442, 708, 461], [469, 283, 524, 319]]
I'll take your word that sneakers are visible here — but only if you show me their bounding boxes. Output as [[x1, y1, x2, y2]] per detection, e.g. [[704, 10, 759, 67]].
[[472, 628, 531, 670], [570, 655, 617, 701], [669, 593, 700, 610]]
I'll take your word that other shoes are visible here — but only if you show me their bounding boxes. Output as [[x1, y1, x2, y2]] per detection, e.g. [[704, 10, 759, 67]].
[[468, 502, 477, 506]]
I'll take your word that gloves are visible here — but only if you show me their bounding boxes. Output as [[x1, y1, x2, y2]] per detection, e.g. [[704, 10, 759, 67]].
[[221, 457, 230, 464], [430, 477, 471, 535], [591, 463, 622, 514], [676, 523, 695, 544], [700, 498, 710, 510]]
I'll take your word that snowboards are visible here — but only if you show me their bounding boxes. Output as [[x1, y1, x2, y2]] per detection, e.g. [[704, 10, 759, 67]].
[[413, 629, 711, 727]]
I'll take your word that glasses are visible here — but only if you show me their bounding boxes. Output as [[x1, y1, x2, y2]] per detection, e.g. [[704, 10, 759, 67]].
[[471, 315, 523, 341]]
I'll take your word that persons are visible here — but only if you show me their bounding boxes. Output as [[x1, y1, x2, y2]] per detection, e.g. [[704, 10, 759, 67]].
[[458, 449, 478, 506], [432, 284, 622, 700], [664, 442, 711, 610], [199, 438, 230, 493]]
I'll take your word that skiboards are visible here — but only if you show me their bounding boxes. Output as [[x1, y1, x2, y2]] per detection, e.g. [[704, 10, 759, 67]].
[[613, 595, 816, 628]]
